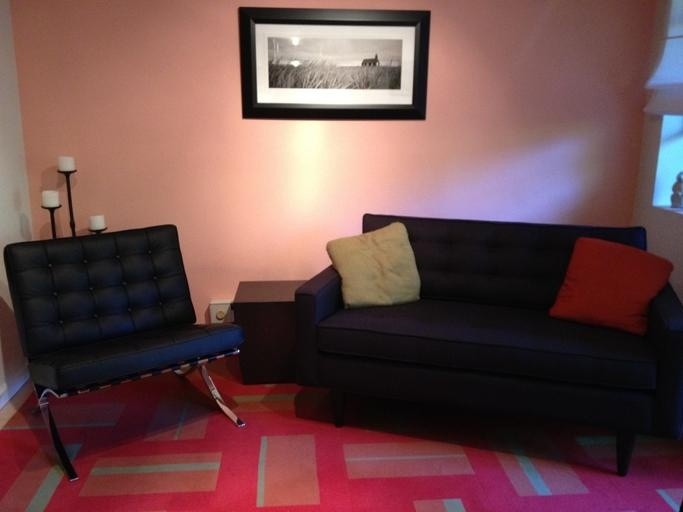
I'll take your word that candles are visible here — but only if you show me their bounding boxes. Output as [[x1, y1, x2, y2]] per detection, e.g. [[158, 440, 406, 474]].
[[57, 157, 75, 171], [41, 190, 59, 207], [89, 215, 106, 229]]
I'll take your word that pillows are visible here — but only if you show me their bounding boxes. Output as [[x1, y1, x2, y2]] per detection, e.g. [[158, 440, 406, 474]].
[[549, 236, 675, 334], [327, 222, 422, 309]]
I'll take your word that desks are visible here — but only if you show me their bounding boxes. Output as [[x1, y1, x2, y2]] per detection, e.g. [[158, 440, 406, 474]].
[[232, 280, 309, 385]]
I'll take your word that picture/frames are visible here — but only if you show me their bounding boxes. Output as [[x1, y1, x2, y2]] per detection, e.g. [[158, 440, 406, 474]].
[[238, 6, 430, 121]]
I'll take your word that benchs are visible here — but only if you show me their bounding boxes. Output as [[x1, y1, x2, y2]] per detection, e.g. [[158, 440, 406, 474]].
[[294, 214, 680, 475], [3, 225, 250, 480]]
